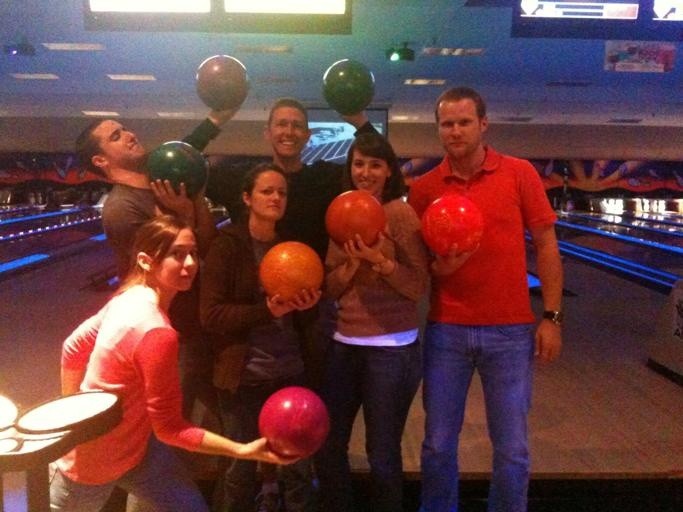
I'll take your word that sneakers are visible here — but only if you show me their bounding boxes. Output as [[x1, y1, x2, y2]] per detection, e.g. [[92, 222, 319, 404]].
[[254, 491, 282, 511]]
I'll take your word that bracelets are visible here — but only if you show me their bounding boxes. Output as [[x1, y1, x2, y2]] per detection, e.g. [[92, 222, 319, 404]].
[[371, 261, 390, 272]]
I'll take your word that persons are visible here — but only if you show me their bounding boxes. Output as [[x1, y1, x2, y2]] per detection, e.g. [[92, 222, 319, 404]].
[[186, 99, 407, 512], [198, 164, 322, 512], [407, 86, 567, 511], [49, 214, 301, 512], [76, 116, 218, 512], [316, 130, 430, 512]]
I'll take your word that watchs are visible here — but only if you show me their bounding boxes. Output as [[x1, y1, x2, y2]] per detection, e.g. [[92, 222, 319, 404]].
[[542, 311, 563, 325]]
